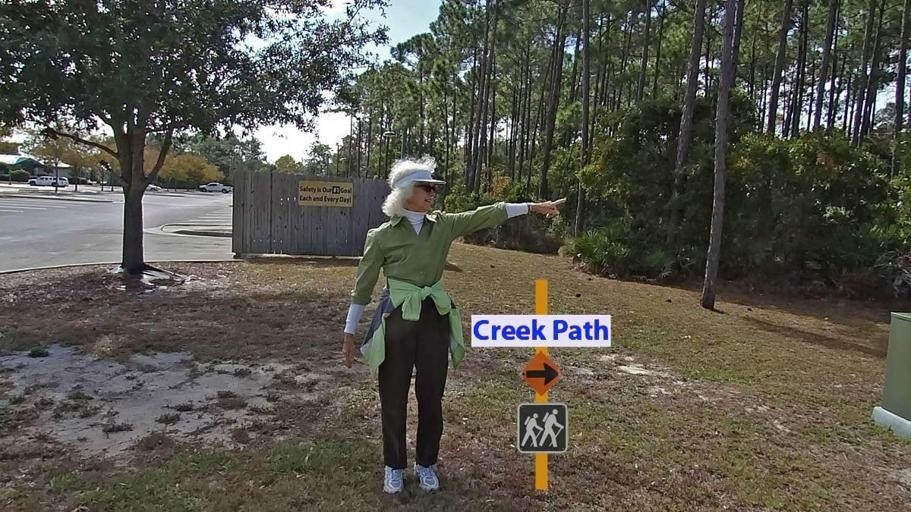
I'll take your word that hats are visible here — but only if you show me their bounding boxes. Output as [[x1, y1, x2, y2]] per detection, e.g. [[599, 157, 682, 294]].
[[395, 171, 446, 186]]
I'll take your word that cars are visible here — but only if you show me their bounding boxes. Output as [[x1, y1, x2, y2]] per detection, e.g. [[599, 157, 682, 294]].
[[146, 184, 161, 191]]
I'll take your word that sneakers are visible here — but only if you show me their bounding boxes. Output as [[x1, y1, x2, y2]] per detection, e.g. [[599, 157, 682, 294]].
[[414, 462, 439, 491], [384, 465, 403, 494]]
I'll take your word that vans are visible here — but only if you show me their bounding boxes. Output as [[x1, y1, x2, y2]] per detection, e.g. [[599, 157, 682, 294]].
[[27, 176, 70, 187]]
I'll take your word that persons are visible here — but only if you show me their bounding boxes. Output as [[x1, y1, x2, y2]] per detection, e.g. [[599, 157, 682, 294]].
[[343, 154, 568, 496]]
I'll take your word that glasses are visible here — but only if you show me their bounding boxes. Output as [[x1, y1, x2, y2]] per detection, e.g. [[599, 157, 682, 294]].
[[416, 184, 436, 192]]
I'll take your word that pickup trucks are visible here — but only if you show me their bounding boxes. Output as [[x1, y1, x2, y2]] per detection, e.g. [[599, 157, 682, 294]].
[[198, 182, 231, 193]]
